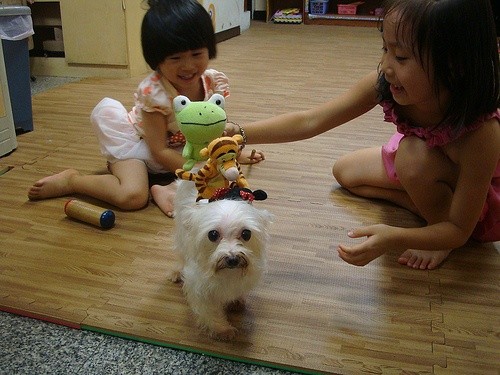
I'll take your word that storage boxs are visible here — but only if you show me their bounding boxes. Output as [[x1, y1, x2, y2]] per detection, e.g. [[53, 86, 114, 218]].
[[338, 2, 365, 14]]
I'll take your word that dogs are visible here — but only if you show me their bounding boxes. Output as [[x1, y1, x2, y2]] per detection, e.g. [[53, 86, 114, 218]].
[[165, 163, 276, 345]]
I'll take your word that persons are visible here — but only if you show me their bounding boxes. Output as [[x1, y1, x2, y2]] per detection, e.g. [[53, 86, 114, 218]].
[[27, 0, 265, 218], [221, 0, 500, 270]]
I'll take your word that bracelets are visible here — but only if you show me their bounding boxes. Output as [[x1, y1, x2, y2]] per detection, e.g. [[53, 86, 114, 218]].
[[227, 120, 247, 150]]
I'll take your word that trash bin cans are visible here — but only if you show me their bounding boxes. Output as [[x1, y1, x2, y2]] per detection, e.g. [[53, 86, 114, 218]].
[[0, 5, 33, 131]]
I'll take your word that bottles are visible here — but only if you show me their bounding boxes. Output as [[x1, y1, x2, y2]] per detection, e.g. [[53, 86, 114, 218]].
[[64, 199, 115, 228]]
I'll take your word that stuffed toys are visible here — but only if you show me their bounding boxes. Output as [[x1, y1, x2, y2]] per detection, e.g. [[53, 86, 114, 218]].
[[174, 134, 250, 202], [172, 93, 227, 171]]
